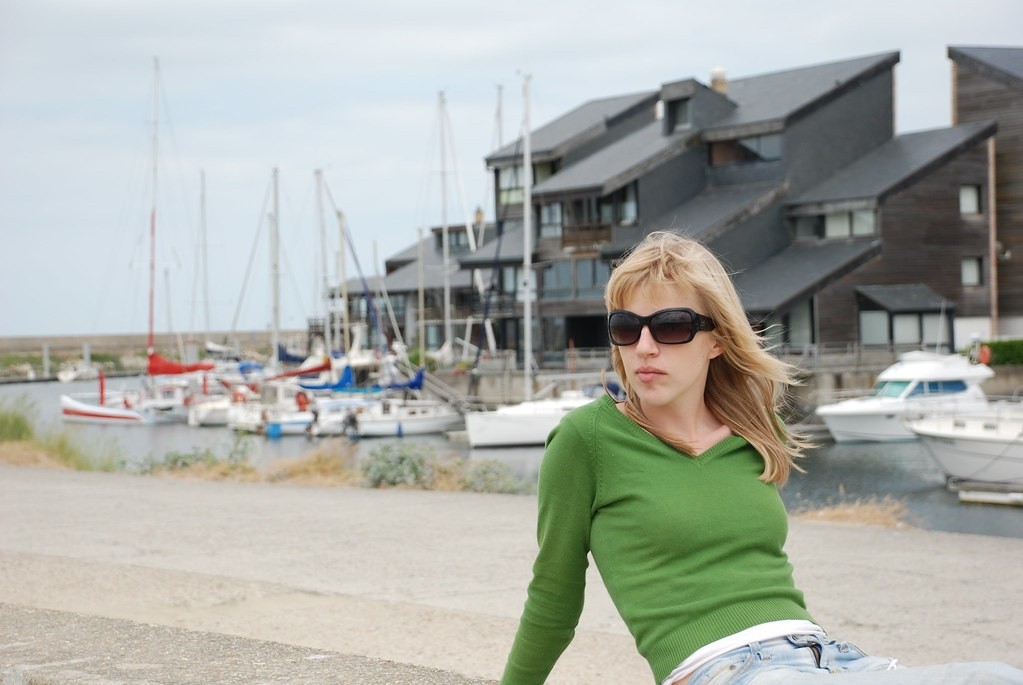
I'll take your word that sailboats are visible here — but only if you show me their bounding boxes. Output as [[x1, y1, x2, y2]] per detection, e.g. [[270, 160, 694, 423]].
[[60, 69, 601, 452]]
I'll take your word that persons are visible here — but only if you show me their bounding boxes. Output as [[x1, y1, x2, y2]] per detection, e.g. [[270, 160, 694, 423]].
[[498, 231, 1023, 685]]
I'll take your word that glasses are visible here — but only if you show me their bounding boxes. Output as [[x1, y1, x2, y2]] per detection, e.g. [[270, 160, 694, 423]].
[[606, 306, 717, 347]]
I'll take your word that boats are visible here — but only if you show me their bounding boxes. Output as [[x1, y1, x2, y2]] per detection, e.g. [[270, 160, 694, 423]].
[[809, 341, 1002, 446], [904, 385, 1022, 510]]
